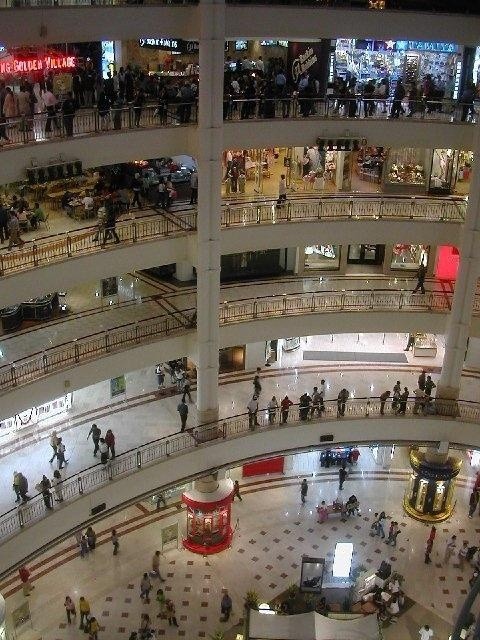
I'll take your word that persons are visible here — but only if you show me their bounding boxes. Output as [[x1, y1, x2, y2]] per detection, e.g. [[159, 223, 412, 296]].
[[18, 563, 35, 596], [110, 529, 121, 556], [468, 471, 480, 520], [224, 57, 478, 123], [128, 551, 179, 640], [1, 159, 199, 252], [74, 524, 98, 559], [346, 495, 357, 517], [232, 481, 242, 501], [417, 624, 434, 640], [221, 592, 232, 623], [316, 500, 328, 524], [87, 422, 114, 468], [1, 61, 200, 143], [221, 146, 288, 210], [370, 512, 399, 547], [156, 359, 191, 432], [352, 447, 361, 465], [300, 479, 308, 504], [11, 430, 69, 511], [247, 367, 436, 429], [423, 526, 479, 587], [411, 264, 426, 294], [338, 464, 346, 491], [64, 595, 100, 640]]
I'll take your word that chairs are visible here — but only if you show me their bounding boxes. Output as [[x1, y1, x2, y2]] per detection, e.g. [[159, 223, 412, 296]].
[[0, 176, 133, 232]]
